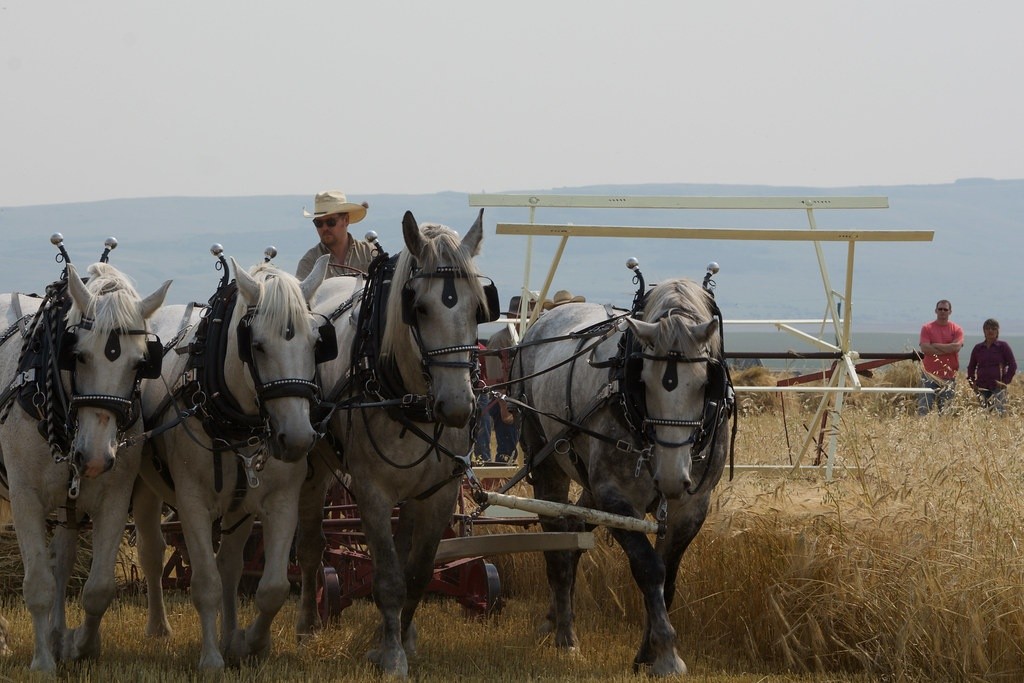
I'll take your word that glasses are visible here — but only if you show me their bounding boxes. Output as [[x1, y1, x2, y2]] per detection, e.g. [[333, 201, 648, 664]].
[[313, 214, 346, 228]]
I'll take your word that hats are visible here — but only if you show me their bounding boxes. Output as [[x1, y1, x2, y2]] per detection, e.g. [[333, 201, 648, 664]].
[[499, 296, 533, 318], [303, 192, 367, 224], [544, 290, 586, 311]]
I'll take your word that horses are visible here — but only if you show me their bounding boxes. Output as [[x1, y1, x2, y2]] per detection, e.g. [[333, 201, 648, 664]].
[[507, 279, 730, 677], [0, 208, 484, 682]]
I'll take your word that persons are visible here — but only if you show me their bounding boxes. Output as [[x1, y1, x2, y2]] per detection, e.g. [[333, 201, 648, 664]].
[[967, 318, 1017, 415], [474, 289, 585, 466], [918, 300, 964, 415], [295, 189, 380, 281]]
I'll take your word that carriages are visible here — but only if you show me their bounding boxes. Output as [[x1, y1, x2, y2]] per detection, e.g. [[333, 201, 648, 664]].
[[2, 207, 739, 683]]
